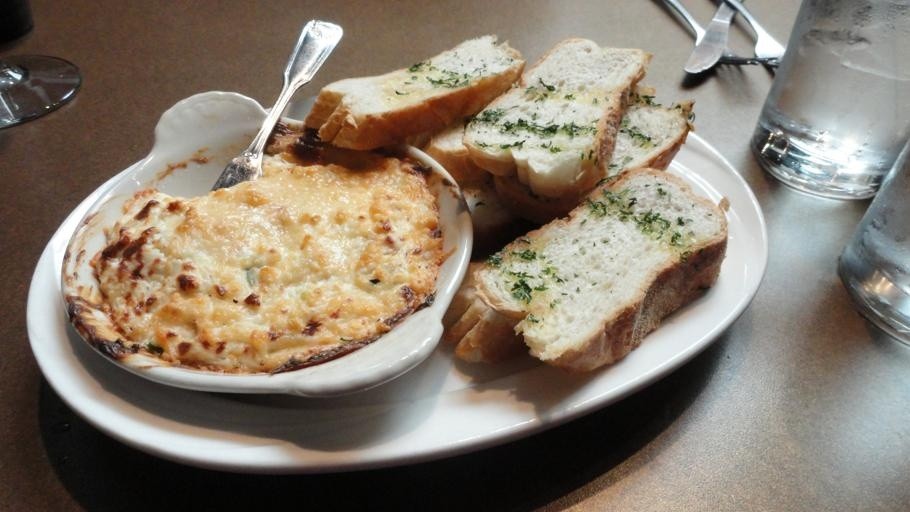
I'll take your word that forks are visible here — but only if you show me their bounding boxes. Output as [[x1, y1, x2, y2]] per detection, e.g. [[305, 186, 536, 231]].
[[211, 19, 343, 193], [654, 0, 786, 68]]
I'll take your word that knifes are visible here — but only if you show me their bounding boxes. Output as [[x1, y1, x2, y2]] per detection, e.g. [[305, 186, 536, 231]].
[[684, 0, 743, 74]]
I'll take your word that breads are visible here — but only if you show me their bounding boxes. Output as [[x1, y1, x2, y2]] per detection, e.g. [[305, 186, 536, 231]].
[[303, 34, 728, 374]]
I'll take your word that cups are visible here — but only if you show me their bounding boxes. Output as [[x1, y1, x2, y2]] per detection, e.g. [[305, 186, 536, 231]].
[[836, 143, 908, 347], [752, 2, 908, 202]]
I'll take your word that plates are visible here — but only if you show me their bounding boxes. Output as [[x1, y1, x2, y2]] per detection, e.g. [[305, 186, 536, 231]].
[[61, 89, 476, 401], [24, 70, 771, 475]]
